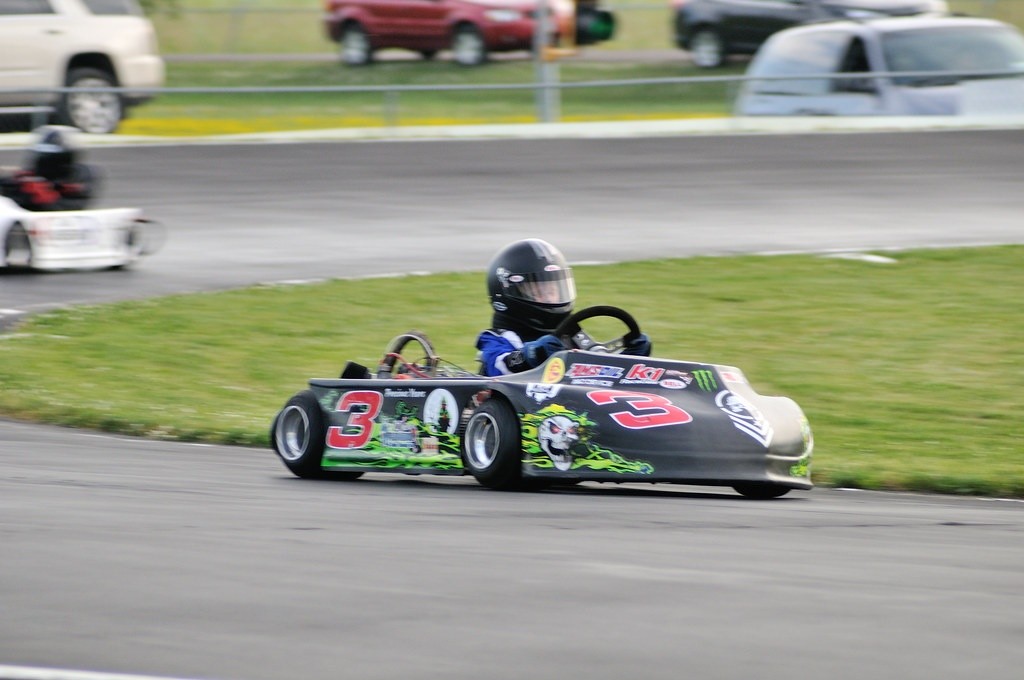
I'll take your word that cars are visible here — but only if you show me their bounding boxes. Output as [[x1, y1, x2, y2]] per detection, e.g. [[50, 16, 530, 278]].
[[323, 0, 562, 67], [670, 0, 949, 69]]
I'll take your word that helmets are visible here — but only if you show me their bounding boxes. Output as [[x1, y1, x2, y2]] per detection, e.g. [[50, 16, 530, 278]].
[[487, 238, 577, 330]]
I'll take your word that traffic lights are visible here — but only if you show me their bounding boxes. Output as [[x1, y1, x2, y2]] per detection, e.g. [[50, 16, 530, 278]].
[[574, 0, 615, 48]]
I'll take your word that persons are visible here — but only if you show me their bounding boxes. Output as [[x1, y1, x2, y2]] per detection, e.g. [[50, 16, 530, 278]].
[[474, 240, 652, 377], [23, 130, 89, 210]]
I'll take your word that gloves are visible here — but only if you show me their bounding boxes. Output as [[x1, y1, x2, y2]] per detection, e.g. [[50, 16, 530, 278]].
[[523, 335, 568, 367], [619, 333, 652, 357]]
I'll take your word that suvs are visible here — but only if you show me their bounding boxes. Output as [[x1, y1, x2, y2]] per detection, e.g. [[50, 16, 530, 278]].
[[0, 0, 164, 137]]
